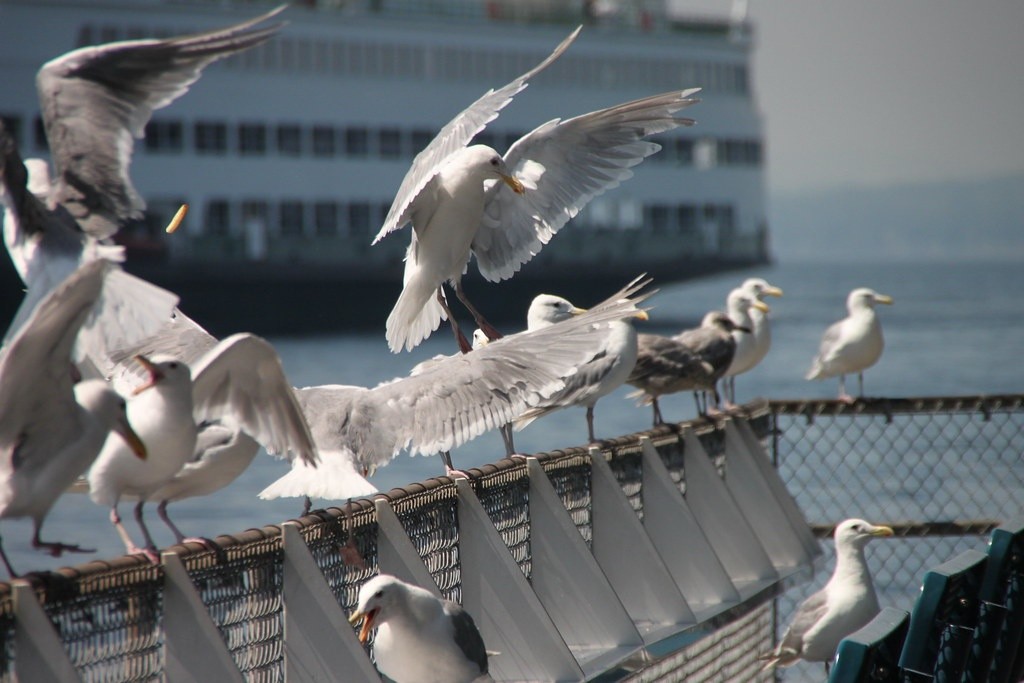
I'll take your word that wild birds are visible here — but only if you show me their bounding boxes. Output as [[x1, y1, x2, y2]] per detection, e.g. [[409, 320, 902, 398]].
[[348, 574, 502, 683], [408, 292, 586, 481], [512, 297, 650, 443], [757, 517, 896, 677], [1, 0, 322, 583], [256, 271, 653, 581], [803, 286, 893, 406], [367, 21, 704, 354], [625, 278, 782, 423]]
